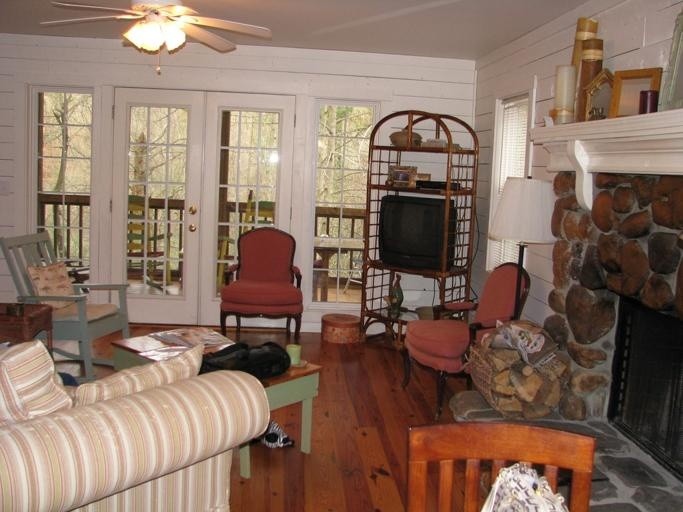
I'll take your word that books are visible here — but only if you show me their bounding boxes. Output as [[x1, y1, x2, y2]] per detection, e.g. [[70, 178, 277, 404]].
[[149, 327, 231, 349]]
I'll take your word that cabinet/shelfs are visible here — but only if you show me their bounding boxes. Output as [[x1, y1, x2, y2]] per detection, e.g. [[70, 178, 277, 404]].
[[358, 108, 482, 351]]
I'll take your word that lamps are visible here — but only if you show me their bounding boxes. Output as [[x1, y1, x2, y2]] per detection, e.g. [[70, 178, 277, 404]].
[[122, 0, 199, 54], [489, 172, 556, 322]]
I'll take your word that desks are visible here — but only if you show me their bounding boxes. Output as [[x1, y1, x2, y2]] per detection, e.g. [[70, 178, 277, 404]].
[[0, 296, 53, 360], [308, 229, 366, 301]]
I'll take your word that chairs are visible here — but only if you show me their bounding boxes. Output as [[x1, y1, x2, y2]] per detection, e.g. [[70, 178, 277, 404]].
[[128, 194, 172, 286], [0, 228, 132, 384], [401, 261, 533, 420], [406, 419, 596, 512], [220, 227, 304, 342]]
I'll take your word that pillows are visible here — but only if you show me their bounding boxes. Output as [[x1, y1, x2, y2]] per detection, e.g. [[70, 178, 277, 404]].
[[72, 341, 206, 405], [0, 339, 73, 422], [26, 259, 77, 309]]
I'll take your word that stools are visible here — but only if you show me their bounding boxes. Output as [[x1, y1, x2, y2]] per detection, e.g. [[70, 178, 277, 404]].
[[320, 313, 363, 345]]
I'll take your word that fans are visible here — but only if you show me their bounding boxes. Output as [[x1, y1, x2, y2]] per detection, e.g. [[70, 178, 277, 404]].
[[37, 0, 274, 52]]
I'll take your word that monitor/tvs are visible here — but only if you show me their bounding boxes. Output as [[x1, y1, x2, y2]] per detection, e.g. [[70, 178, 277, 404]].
[[379, 195, 458, 271]]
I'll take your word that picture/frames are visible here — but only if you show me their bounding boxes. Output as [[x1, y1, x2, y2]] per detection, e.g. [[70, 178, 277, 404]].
[[655, 10, 683, 111], [610, 68, 664, 120], [582, 68, 621, 121]]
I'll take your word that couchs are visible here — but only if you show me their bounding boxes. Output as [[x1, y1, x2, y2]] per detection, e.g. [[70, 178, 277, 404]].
[[0, 334, 269, 512]]
